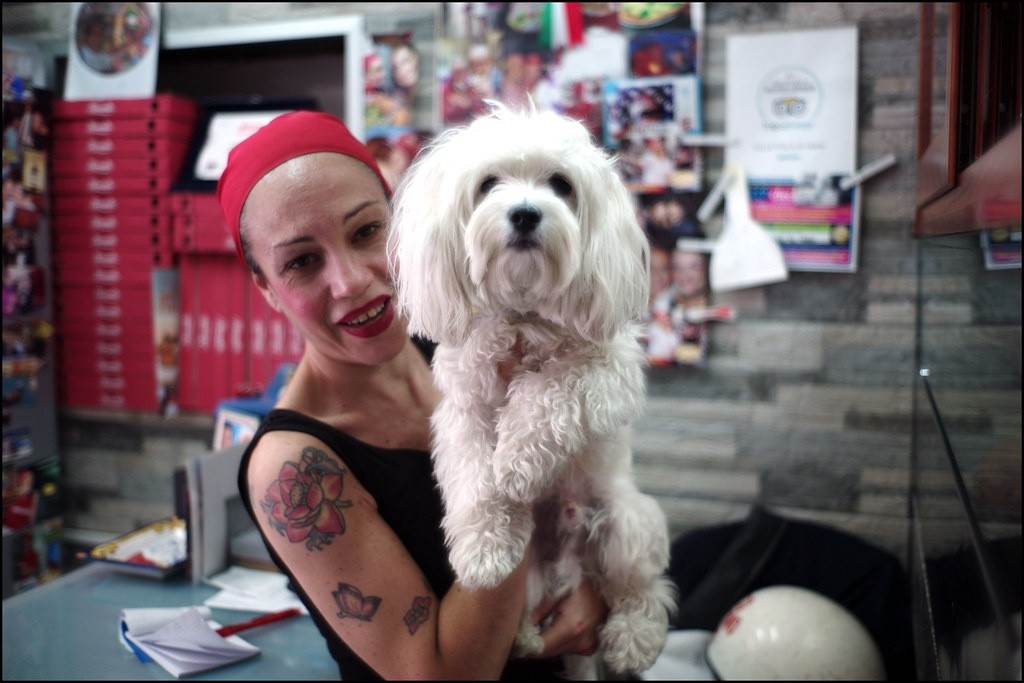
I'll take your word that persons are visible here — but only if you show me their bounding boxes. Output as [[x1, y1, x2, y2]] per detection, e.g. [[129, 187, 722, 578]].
[[363, 37, 419, 129], [467, 37, 547, 114], [217, 110, 611, 680], [621, 91, 709, 369], [75, 13, 138, 76]]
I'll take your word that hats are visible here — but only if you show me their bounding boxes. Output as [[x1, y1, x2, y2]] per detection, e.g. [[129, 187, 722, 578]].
[[213, 108, 395, 267]]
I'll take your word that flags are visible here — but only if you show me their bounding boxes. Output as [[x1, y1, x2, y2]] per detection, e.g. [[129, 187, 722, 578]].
[[541, 3, 585, 47]]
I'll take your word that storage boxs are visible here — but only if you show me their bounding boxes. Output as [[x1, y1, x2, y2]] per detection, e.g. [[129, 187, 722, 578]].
[[53, 95, 306, 414]]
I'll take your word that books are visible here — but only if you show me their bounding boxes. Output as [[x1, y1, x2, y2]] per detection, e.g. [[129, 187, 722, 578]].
[[116, 610, 259, 680]]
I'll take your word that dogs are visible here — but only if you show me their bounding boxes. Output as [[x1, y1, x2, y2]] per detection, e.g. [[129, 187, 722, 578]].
[[385, 88, 680, 681]]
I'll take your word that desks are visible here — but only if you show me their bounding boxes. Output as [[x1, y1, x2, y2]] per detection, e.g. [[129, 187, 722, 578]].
[[0, 562, 342, 679]]
